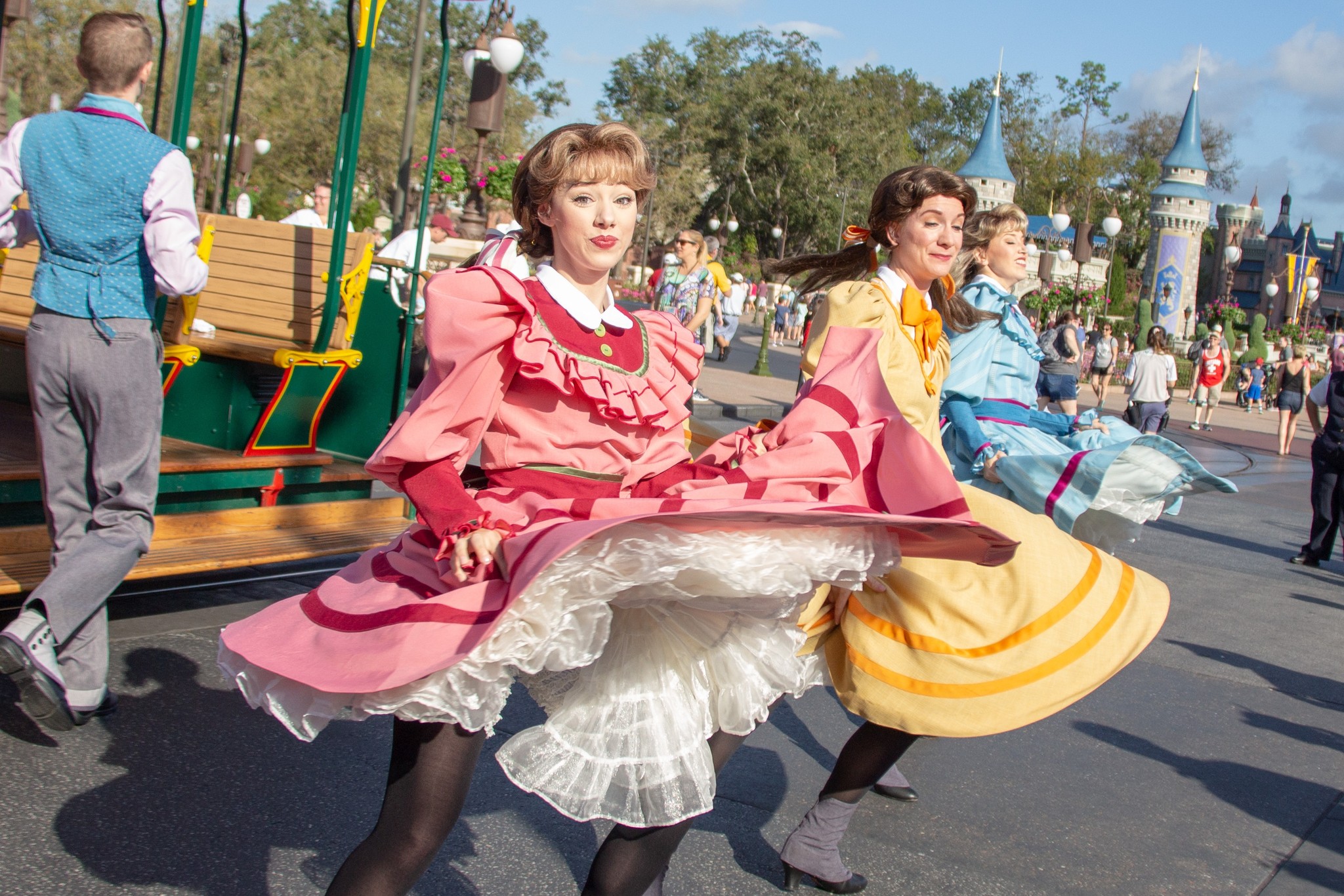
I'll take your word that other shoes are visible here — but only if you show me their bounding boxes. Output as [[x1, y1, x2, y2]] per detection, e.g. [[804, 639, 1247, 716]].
[[1075, 384, 1278, 431], [718, 346, 732, 362], [742, 309, 767, 315], [770, 333, 804, 357]]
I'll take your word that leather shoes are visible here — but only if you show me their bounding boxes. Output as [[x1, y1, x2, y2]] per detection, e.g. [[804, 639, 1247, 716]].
[[1290, 549, 1321, 566], [0, 635, 75, 731], [70, 688, 119, 726]]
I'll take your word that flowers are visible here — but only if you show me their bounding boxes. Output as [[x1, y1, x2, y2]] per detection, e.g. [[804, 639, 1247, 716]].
[[1024, 276, 1113, 312], [410, 146, 529, 203], [1201, 297, 1329, 345]]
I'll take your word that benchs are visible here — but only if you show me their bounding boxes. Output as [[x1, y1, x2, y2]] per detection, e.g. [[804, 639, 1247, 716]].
[[162, 210, 379, 457], [2, 211, 217, 442]]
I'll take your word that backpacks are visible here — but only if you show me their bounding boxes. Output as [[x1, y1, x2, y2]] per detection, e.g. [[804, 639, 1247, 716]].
[[1036, 329, 1060, 367], [1187, 339, 1204, 360]]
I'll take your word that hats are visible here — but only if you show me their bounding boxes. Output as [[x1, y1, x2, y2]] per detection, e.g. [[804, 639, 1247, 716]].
[[1209, 331, 1221, 340], [431, 214, 459, 238], [1093, 323, 1099, 329], [664, 253, 678, 264], [731, 272, 743, 282], [1211, 324, 1222, 332], [1254, 357, 1264, 366]]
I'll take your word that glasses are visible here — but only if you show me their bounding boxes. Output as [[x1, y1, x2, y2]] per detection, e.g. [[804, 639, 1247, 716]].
[[1102, 328, 1111, 331], [313, 195, 331, 200], [1074, 319, 1082, 327], [676, 239, 697, 246]]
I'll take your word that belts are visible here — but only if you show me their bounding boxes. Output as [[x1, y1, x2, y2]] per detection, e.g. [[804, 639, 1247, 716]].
[[722, 313, 739, 317]]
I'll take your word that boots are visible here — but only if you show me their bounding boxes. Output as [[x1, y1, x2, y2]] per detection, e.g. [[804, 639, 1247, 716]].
[[780, 790, 869, 896], [874, 764, 919, 802]]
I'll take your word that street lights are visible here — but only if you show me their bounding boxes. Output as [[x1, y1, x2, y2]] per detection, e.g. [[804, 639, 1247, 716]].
[[1266, 264, 1322, 348], [1051, 184, 1122, 315], [772, 208, 789, 283], [455, 0, 525, 236], [1027, 225, 1073, 332], [1222, 230, 1241, 305], [708, 183, 741, 262]]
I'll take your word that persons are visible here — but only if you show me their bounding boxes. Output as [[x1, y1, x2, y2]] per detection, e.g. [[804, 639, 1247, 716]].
[[649, 227, 716, 417], [609, 228, 828, 350], [278, 182, 355, 234], [367, 210, 461, 324], [1026, 308, 1343, 457], [757, 166, 1013, 894], [1287, 344, 1344, 568], [324, 123, 761, 896], [0, 11, 213, 735], [935, 203, 1110, 512], [693, 235, 734, 402]]
